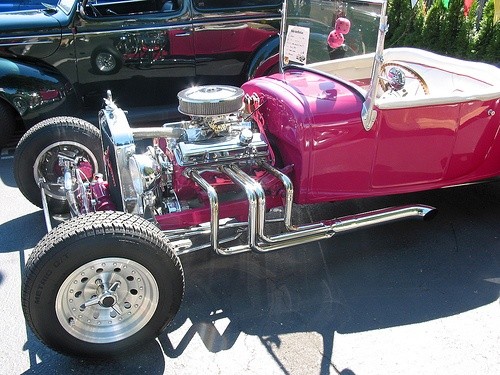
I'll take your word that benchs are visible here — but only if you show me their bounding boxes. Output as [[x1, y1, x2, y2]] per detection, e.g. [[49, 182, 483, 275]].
[[390, 57, 493, 99]]
